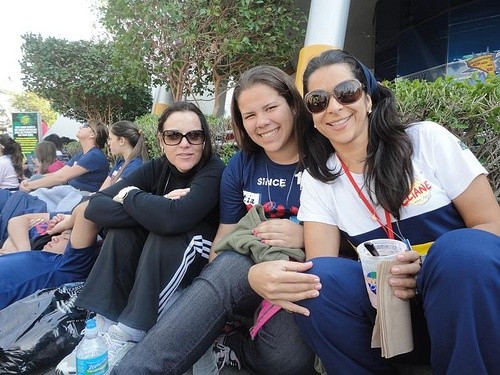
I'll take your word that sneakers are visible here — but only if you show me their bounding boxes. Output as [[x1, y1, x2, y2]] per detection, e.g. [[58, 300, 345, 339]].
[[212, 331, 242, 372], [62, 325, 105, 374], [103, 325, 137, 375]]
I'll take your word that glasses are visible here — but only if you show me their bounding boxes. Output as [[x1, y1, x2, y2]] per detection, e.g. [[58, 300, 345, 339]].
[[159, 129, 207, 146], [82, 123, 95, 134]]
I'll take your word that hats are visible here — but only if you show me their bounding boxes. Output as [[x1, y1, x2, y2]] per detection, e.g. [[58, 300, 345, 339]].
[[303, 79, 365, 115]]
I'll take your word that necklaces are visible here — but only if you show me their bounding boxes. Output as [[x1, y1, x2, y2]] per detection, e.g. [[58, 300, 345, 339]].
[[336, 153, 395, 239], [264, 158, 300, 213]]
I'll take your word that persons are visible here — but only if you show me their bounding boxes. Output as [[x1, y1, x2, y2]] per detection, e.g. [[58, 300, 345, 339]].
[[111, 65, 342, 375], [56, 101, 226, 375], [249, 49, 499, 375], [0, 119, 147, 310]]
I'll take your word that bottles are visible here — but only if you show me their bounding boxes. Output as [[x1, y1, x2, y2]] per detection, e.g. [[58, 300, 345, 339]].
[[75, 318, 110, 375]]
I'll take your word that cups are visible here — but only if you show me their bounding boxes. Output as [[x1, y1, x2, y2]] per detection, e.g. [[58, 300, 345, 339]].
[[356, 238, 408, 310]]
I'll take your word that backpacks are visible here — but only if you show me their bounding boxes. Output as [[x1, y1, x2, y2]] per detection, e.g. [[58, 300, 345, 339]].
[[0, 191, 47, 247]]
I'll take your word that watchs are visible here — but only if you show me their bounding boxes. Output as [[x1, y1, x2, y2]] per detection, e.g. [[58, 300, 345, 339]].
[[118, 186, 139, 204]]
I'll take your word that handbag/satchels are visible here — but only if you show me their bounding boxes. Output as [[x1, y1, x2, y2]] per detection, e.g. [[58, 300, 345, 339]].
[[0, 281, 97, 374]]
